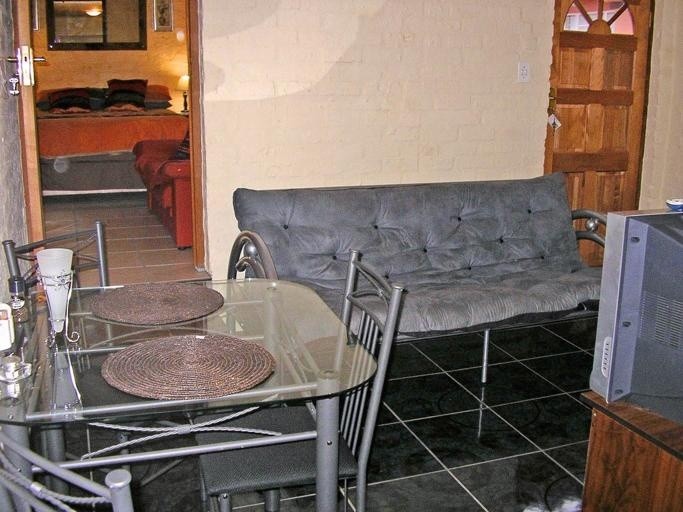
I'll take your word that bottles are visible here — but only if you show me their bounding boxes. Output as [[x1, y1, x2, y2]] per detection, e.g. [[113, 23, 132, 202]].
[[0, 277, 29, 353]]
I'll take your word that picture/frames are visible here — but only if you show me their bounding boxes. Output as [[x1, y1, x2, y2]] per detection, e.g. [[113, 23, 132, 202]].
[[154, 0, 173, 32], [32, 0, 38, 30]]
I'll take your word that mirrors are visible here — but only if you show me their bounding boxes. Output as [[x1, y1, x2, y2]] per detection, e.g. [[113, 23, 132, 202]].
[[45, 0, 147, 50]]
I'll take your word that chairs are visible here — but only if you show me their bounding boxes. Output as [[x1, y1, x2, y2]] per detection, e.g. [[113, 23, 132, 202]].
[[2, 222, 166, 473], [132, 132, 192, 248], [189, 248, 406, 512], [0, 396, 134, 512]]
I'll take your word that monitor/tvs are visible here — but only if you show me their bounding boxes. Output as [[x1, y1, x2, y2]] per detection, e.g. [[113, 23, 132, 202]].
[[588, 207, 683, 424]]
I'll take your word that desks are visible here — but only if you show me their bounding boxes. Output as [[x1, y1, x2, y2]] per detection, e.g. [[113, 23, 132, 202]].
[[579, 392, 683, 512]]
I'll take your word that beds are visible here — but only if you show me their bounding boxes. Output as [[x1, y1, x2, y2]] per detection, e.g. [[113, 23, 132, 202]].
[[34, 85, 190, 196]]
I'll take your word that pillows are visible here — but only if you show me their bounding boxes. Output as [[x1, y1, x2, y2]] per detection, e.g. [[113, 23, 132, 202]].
[[175, 131, 190, 160], [35, 79, 172, 113]]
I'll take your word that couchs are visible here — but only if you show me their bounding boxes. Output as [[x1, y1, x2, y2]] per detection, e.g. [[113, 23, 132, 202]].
[[225, 172, 607, 383]]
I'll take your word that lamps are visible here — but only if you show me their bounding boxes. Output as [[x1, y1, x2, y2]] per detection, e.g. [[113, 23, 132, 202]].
[[174, 76, 189, 112], [83, 9, 103, 17]]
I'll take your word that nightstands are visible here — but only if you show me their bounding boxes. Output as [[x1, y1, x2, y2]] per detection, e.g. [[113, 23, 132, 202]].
[[176, 112, 188, 117]]
